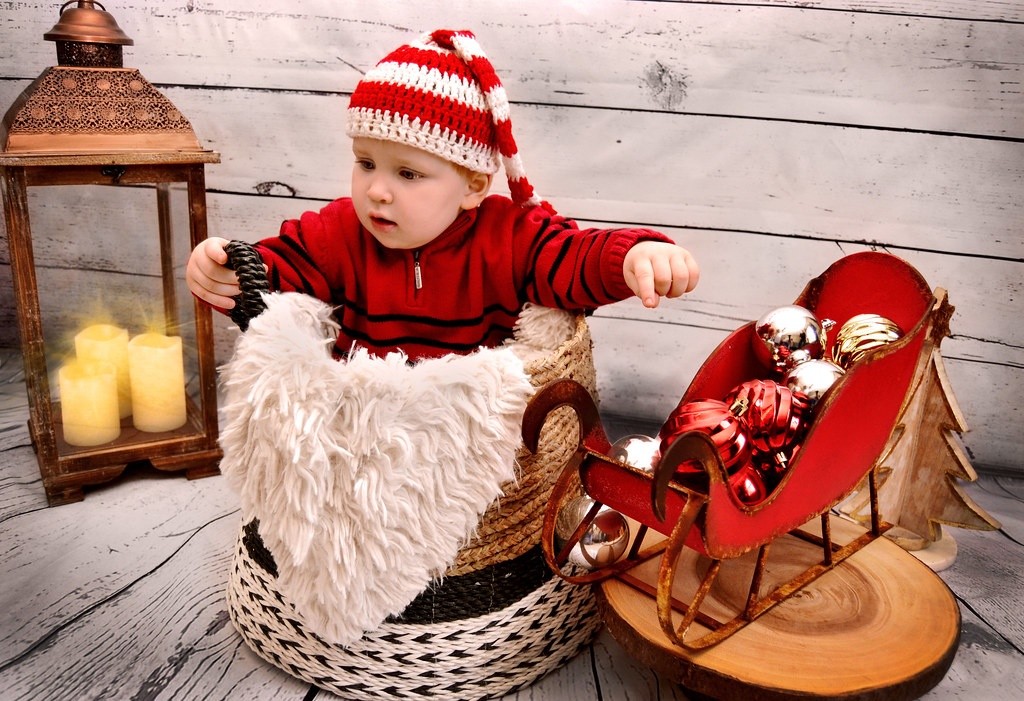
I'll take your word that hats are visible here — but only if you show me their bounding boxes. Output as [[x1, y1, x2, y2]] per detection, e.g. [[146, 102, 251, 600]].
[[343, 29, 558, 217]]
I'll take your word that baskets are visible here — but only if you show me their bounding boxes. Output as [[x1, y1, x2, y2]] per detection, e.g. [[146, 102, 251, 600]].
[[215, 235, 603, 700]]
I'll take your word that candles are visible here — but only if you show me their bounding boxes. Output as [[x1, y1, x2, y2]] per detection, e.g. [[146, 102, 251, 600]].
[[128, 334, 187, 433], [75, 325, 133, 421], [59, 360, 121, 447]]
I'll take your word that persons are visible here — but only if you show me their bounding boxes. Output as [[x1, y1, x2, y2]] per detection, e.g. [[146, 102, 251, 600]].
[[185, 28, 699, 365]]
